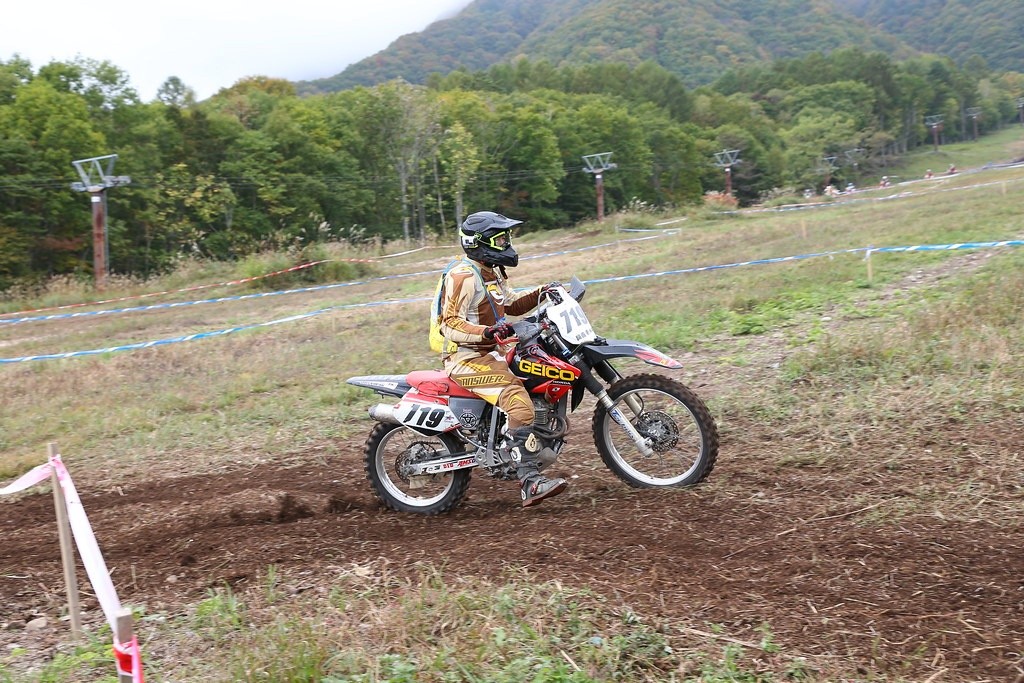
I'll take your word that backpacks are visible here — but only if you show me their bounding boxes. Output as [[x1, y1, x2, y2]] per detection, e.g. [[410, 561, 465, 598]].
[[429, 254, 486, 354]]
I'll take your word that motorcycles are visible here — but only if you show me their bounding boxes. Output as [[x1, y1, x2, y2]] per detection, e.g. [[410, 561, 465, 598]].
[[342, 275, 721, 510]]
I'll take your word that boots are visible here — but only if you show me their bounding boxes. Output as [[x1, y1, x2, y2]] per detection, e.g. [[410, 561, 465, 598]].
[[505, 425, 567, 507]]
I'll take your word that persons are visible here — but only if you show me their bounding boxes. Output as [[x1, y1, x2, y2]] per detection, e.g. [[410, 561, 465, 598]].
[[879, 176, 891, 189], [429, 211, 568, 507], [804, 181, 858, 200], [947, 163, 958, 174], [925, 170, 934, 180]]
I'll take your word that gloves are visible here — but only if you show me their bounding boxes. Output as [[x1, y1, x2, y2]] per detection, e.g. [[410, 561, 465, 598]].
[[542, 281, 568, 305], [482, 321, 515, 342]]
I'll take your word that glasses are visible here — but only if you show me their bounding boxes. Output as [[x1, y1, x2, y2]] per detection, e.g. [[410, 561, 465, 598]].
[[460, 227, 512, 251]]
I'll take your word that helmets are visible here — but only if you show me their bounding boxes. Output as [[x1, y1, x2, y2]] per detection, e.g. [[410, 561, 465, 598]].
[[461, 211, 523, 267]]
